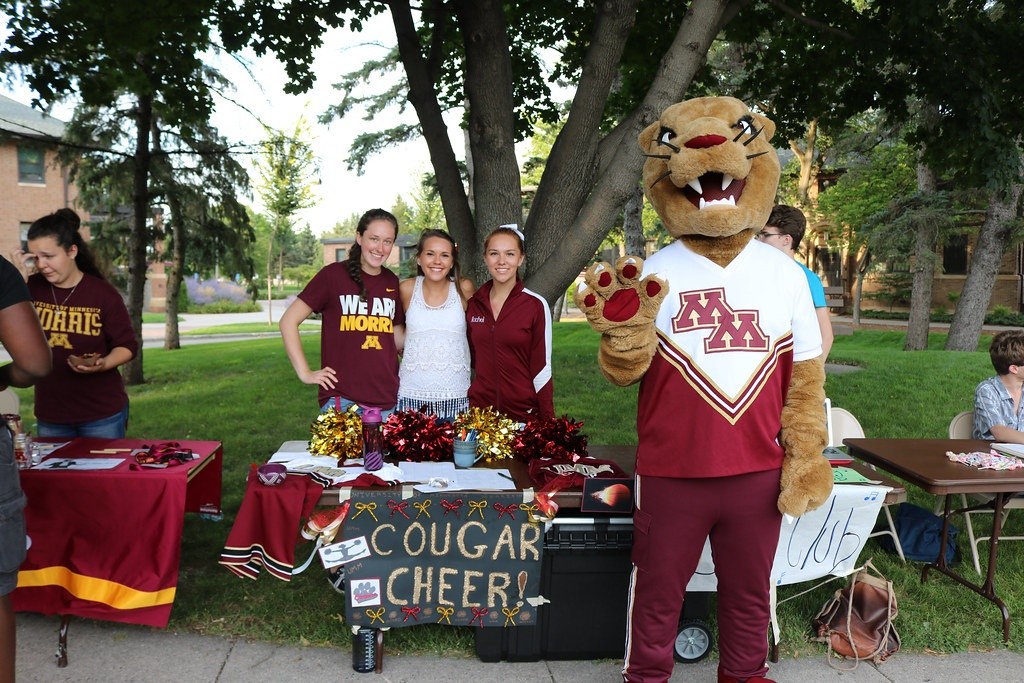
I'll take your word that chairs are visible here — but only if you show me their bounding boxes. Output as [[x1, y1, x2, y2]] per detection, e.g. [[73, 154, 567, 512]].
[[829, 408, 907, 566], [929, 410, 1024, 576]]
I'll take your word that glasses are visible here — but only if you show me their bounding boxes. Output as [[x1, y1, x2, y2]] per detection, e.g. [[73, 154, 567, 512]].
[[757, 231, 782, 238]]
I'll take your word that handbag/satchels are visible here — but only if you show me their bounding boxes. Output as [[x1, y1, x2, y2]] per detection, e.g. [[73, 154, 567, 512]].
[[813, 561, 901, 670]]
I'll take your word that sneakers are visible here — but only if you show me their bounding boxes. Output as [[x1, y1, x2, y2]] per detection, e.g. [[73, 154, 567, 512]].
[[326, 566, 345, 595]]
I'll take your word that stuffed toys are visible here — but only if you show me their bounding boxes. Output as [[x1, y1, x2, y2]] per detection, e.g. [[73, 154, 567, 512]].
[[574, 96, 834, 683]]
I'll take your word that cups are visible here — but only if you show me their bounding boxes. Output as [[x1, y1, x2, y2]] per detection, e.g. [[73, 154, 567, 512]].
[[453, 437, 486, 467]]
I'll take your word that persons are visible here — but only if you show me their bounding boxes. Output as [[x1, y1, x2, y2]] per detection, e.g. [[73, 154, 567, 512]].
[[399, 229, 474, 423], [758, 205, 834, 363], [279, 209, 407, 424], [972, 329, 1024, 444], [466, 224, 554, 424], [0, 255, 52, 683], [10, 208, 138, 439]]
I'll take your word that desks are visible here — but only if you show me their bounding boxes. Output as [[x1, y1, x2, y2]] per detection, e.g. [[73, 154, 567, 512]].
[[220, 442, 907, 673], [11, 437, 223, 667], [842, 438, 1024, 644]]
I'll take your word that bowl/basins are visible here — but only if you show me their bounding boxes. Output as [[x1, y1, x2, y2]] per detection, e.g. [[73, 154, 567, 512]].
[[257, 464, 287, 486]]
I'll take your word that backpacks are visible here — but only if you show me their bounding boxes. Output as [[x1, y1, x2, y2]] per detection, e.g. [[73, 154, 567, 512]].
[[877, 503, 959, 565]]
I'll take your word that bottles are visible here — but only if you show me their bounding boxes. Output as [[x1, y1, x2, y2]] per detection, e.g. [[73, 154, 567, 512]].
[[362, 408, 383, 471], [2, 413, 41, 470]]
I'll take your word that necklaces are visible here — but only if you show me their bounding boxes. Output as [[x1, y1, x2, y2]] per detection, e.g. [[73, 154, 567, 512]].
[[52, 286, 77, 315]]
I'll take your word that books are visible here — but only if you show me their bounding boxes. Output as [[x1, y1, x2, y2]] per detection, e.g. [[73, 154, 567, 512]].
[[990, 443, 1024, 458]]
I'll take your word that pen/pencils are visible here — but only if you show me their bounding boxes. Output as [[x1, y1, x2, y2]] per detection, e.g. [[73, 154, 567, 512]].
[[104, 449, 133, 452], [499, 473, 516, 483], [462, 427, 478, 441], [90, 449, 117, 454]]
